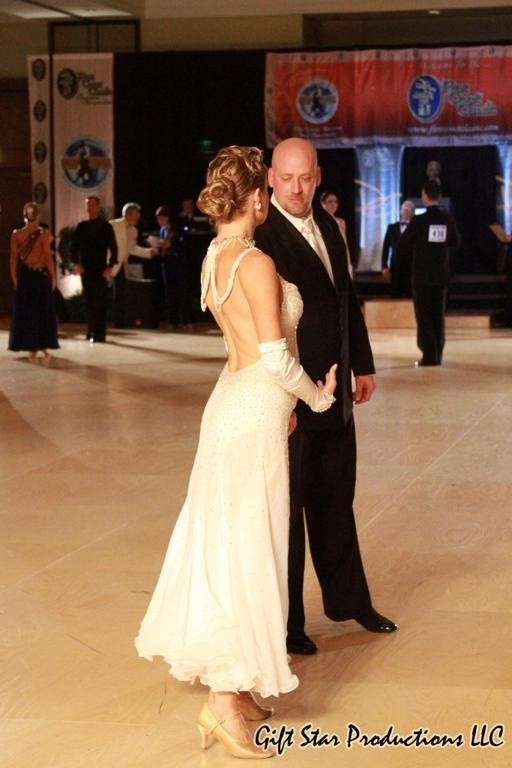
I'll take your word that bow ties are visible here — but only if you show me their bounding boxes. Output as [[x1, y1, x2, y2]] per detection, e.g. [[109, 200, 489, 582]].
[[400, 222, 409, 226]]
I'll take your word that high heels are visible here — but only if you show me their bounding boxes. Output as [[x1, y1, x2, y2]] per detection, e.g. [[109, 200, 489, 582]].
[[196, 697, 275, 758]]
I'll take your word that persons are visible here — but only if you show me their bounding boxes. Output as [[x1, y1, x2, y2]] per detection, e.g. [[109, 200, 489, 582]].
[[106, 200, 215, 329], [9, 203, 56, 363], [69, 196, 119, 342], [380, 200, 416, 300], [424, 160, 451, 215], [319, 191, 354, 282], [136, 146, 337, 758], [401, 179, 462, 366], [253, 136, 399, 656]]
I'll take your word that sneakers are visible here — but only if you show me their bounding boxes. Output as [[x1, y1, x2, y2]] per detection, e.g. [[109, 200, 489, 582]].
[[415, 358, 439, 366]]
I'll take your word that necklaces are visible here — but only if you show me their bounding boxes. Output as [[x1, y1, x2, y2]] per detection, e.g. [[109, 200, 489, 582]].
[[207, 232, 258, 254]]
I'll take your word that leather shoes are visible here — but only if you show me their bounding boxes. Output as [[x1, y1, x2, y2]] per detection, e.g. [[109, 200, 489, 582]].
[[355, 607, 398, 631], [286, 632, 318, 654]]
